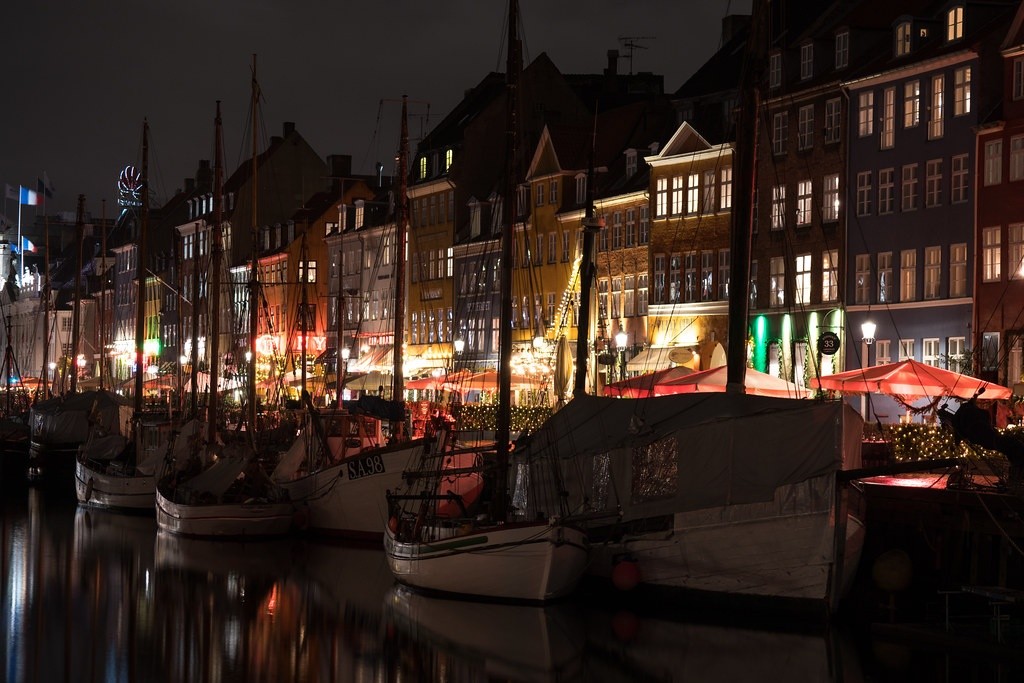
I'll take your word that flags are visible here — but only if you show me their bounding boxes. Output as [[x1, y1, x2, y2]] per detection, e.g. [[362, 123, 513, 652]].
[[22, 235, 38, 254], [20, 187, 44, 205], [10, 244, 21, 252]]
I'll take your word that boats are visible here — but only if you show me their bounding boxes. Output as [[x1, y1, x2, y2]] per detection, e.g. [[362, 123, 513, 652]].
[[28, 486, 865, 683]]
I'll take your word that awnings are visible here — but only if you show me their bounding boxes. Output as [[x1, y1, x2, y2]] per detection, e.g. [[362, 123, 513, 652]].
[[349, 343, 456, 378]]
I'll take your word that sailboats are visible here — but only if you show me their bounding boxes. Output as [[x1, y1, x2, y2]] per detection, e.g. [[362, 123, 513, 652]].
[[0, 0, 866, 622]]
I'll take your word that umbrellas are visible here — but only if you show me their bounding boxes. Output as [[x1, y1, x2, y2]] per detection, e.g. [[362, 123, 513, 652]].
[[654, 360, 815, 398], [822, 358, 1012, 425], [406, 369, 486, 392], [602, 364, 698, 396], [7, 374, 245, 392], [442, 367, 550, 402]]
